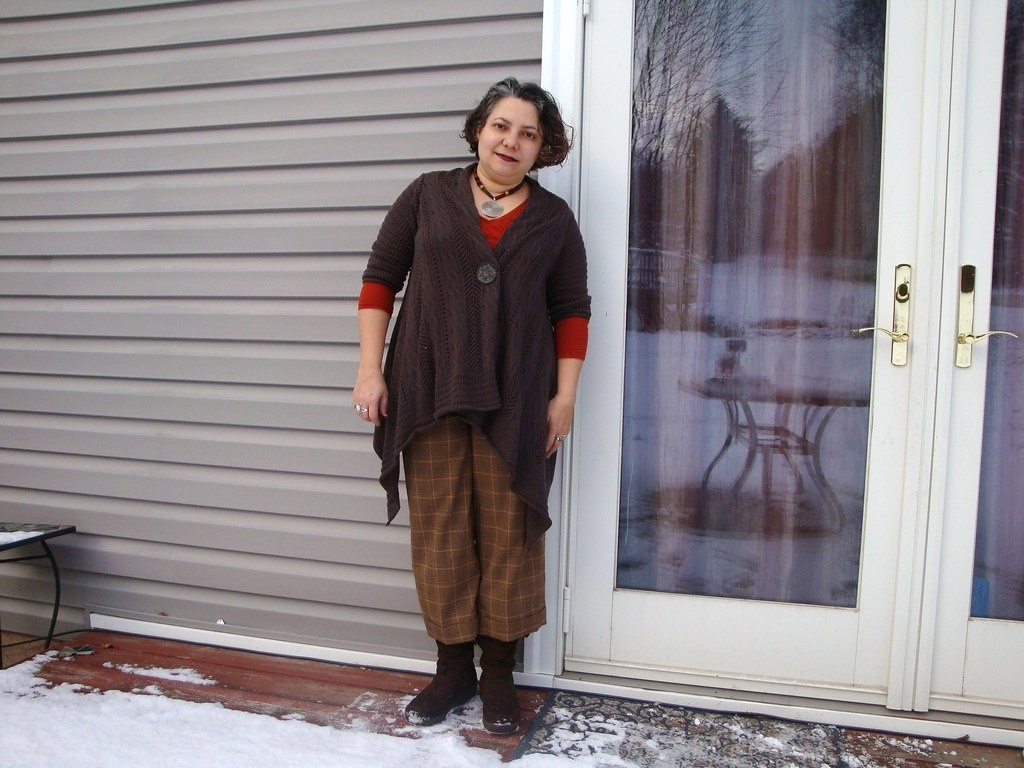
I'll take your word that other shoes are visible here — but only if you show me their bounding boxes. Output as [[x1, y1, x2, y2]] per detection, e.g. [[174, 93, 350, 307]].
[[479, 658, 520, 733], [405, 660, 478, 726]]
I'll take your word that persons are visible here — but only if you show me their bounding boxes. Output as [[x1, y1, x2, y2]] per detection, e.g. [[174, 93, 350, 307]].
[[352, 77, 591, 734]]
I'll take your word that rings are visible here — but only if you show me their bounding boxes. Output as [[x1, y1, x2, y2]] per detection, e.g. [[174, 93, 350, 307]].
[[355, 405, 360, 410], [563, 435, 567, 438], [556, 438, 562, 441], [361, 406, 368, 413], [352, 401, 355, 403]]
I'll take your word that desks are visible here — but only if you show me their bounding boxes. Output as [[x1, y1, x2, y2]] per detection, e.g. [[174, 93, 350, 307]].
[[678, 372, 869, 546]]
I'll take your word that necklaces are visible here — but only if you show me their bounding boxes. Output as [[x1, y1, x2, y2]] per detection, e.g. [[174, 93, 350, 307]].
[[473, 166, 525, 219]]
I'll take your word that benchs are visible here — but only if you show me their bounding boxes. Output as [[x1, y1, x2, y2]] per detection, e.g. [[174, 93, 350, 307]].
[[0, 520, 75, 669]]
